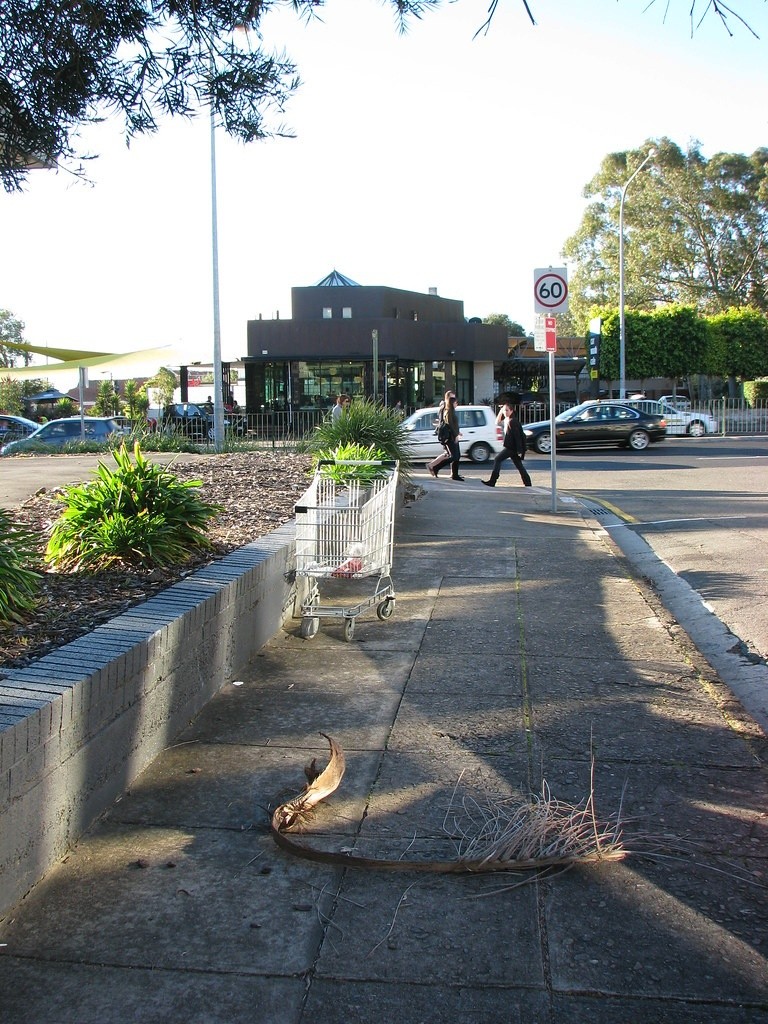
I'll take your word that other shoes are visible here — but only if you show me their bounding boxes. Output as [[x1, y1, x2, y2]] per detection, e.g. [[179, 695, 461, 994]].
[[425, 461, 435, 476], [481, 480, 494, 487], [432, 465, 439, 479], [451, 476, 464, 481]]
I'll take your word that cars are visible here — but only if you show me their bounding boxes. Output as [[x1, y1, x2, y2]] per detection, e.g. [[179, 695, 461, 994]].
[[522, 404, 667, 453], [1, 418, 121, 455], [397, 406, 504, 463], [0, 415, 42, 444], [521, 400, 545, 418], [659, 395, 690, 408], [584, 395, 717, 437], [160, 402, 247, 441]]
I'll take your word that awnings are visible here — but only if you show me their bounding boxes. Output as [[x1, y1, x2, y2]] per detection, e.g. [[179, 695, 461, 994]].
[[20, 388, 80, 422]]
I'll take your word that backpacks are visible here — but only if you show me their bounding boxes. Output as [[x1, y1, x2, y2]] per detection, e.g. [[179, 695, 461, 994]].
[[437, 420, 452, 445]]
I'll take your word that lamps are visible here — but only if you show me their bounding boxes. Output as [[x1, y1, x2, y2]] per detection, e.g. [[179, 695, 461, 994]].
[[261, 349, 269, 356], [449, 350, 456, 357]]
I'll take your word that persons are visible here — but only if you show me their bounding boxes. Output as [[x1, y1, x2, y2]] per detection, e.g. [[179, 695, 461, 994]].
[[393, 400, 403, 414], [205, 396, 213, 414], [228, 391, 234, 408], [582, 408, 597, 422], [427, 389, 463, 478], [0, 421, 10, 437], [266, 379, 369, 423], [331, 395, 350, 435], [480, 402, 533, 487], [429, 397, 465, 482], [232, 401, 243, 437]]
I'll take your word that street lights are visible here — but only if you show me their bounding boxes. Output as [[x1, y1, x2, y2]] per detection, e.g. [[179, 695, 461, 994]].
[[208, 15, 252, 453], [619, 149, 660, 399]]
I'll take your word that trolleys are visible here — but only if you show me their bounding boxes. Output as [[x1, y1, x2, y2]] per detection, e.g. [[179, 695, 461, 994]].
[[292, 459, 401, 641]]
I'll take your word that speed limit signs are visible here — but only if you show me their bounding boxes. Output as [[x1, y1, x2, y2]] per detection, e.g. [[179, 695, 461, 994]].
[[533, 267, 569, 313]]
